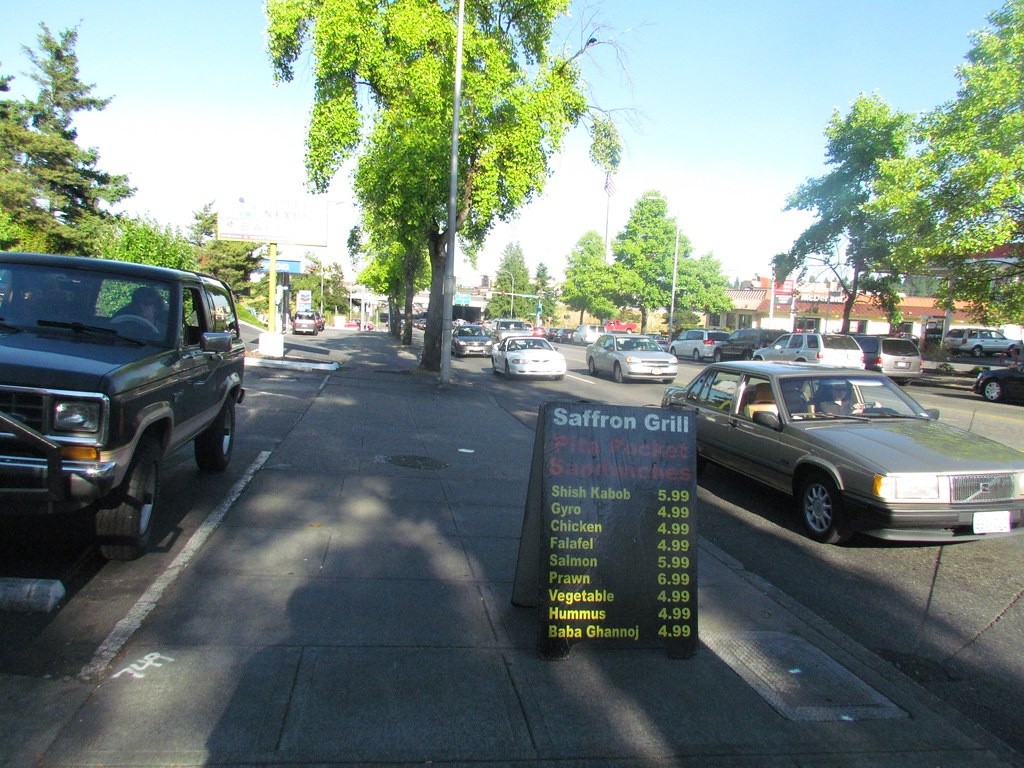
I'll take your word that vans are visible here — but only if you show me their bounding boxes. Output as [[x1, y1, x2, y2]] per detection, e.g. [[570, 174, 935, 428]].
[[491, 318, 534, 345], [572, 324, 606, 346]]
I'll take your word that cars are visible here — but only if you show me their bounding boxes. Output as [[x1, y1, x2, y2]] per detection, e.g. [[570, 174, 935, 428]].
[[586, 334, 678, 384], [451, 325, 494, 358], [412, 319, 427, 330], [661, 361, 1024, 544], [525, 323, 574, 344], [973, 361, 1024, 407], [492, 336, 566, 380]]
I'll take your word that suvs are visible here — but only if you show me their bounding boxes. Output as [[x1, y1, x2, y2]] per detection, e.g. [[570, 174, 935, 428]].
[[943, 328, 1020, 359], [0, 253, 246, 561], [669, 328, 923, 386], [291, 309, 326, 336]]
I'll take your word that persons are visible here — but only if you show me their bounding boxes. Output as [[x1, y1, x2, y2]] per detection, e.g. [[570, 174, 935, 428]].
[[131, 287, 167, 334], [819, 383, 862, 414], [357, 319, 361, 329]]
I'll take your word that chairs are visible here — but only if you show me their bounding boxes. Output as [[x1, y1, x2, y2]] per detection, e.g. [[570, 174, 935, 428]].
[[509, 344, 516, 349], [745, 383, 779, 419]]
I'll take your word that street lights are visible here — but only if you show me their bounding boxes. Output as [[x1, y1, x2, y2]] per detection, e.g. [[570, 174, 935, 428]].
[[494, 267, 515, 322], [646, 193, 682, 345]]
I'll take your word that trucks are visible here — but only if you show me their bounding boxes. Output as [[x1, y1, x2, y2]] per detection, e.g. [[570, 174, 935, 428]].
[[604, 319, 638, 334]]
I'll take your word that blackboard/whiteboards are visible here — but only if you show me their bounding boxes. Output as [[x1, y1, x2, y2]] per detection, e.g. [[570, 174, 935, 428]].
[[512, 400, 699, 645]]
